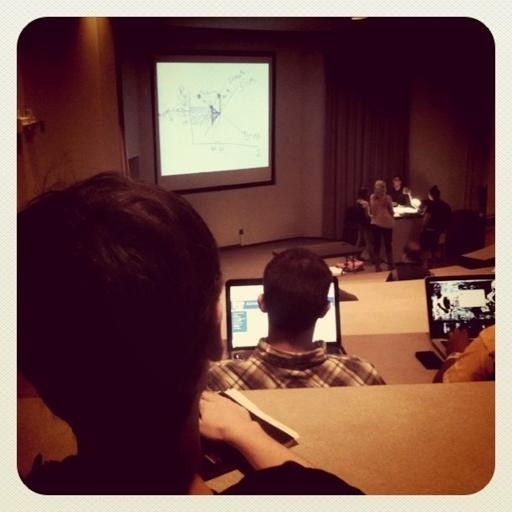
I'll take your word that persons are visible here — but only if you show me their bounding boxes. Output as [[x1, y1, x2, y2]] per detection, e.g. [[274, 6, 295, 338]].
[[17, 168, 368, 495], [202, 247, 386, 393], [432, 279, 495, 385], [347, 168, 450, 272]]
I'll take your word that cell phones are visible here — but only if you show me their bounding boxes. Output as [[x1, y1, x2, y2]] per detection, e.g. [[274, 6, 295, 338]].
[[415, 350, 444, 370]]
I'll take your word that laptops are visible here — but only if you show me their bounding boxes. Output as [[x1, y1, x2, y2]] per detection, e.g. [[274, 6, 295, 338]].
[[225, 276, 348, 360], [425, 274, 495, 361]]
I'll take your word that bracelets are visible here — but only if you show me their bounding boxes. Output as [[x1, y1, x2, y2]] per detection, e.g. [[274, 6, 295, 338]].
[[445, 352, 463, 361]]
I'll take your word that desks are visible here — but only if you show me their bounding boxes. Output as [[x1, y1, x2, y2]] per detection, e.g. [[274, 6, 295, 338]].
[[17, 245, 495, 495]]
